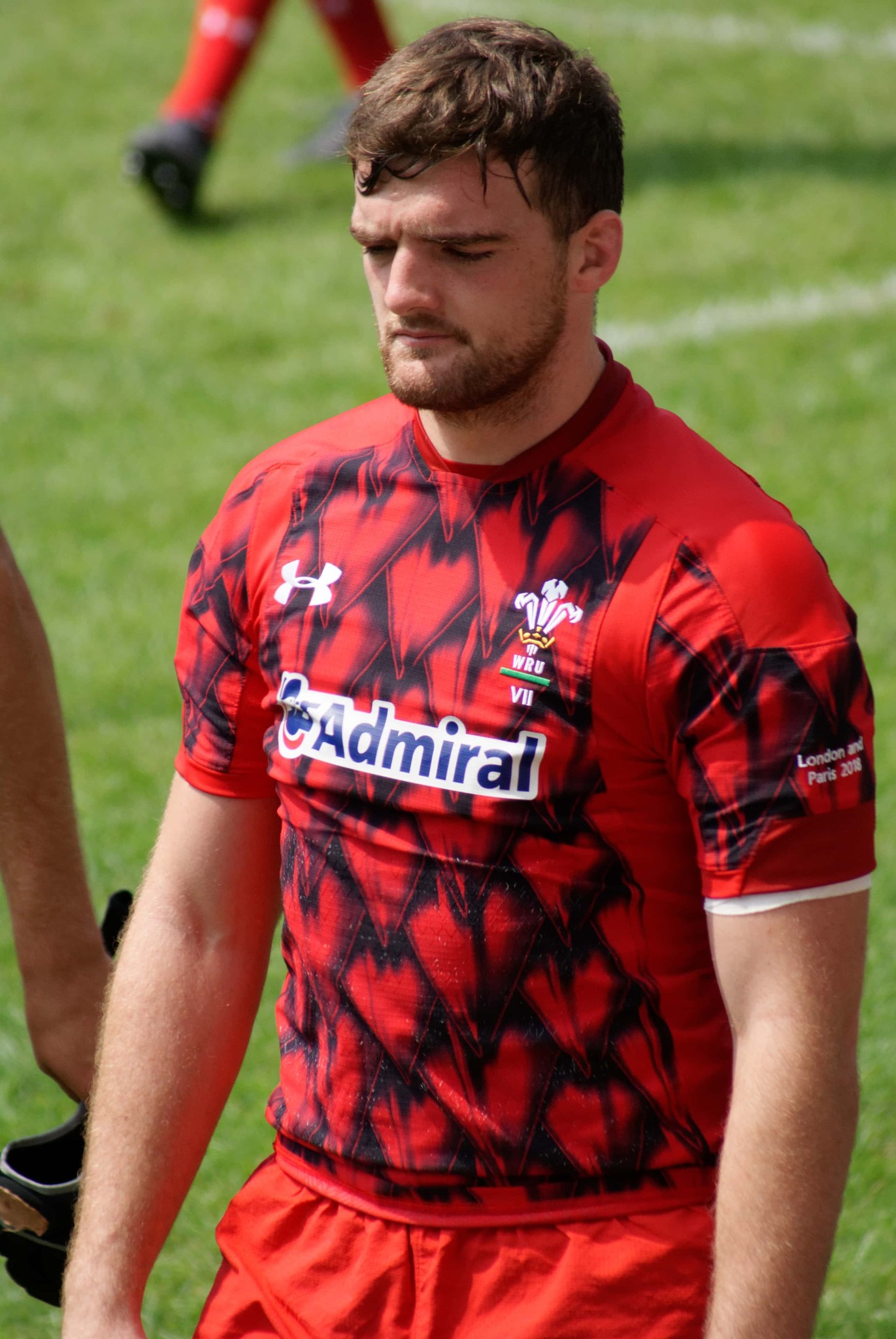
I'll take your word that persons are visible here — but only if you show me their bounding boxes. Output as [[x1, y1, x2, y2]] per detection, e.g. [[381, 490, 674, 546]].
[[0, 533, 136, 1111], [58, 14, 875, 1339], [128, 0, 396, 224]]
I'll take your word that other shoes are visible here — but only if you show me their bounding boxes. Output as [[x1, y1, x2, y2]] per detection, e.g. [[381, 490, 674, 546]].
[[134, 120, 215, 229]]
